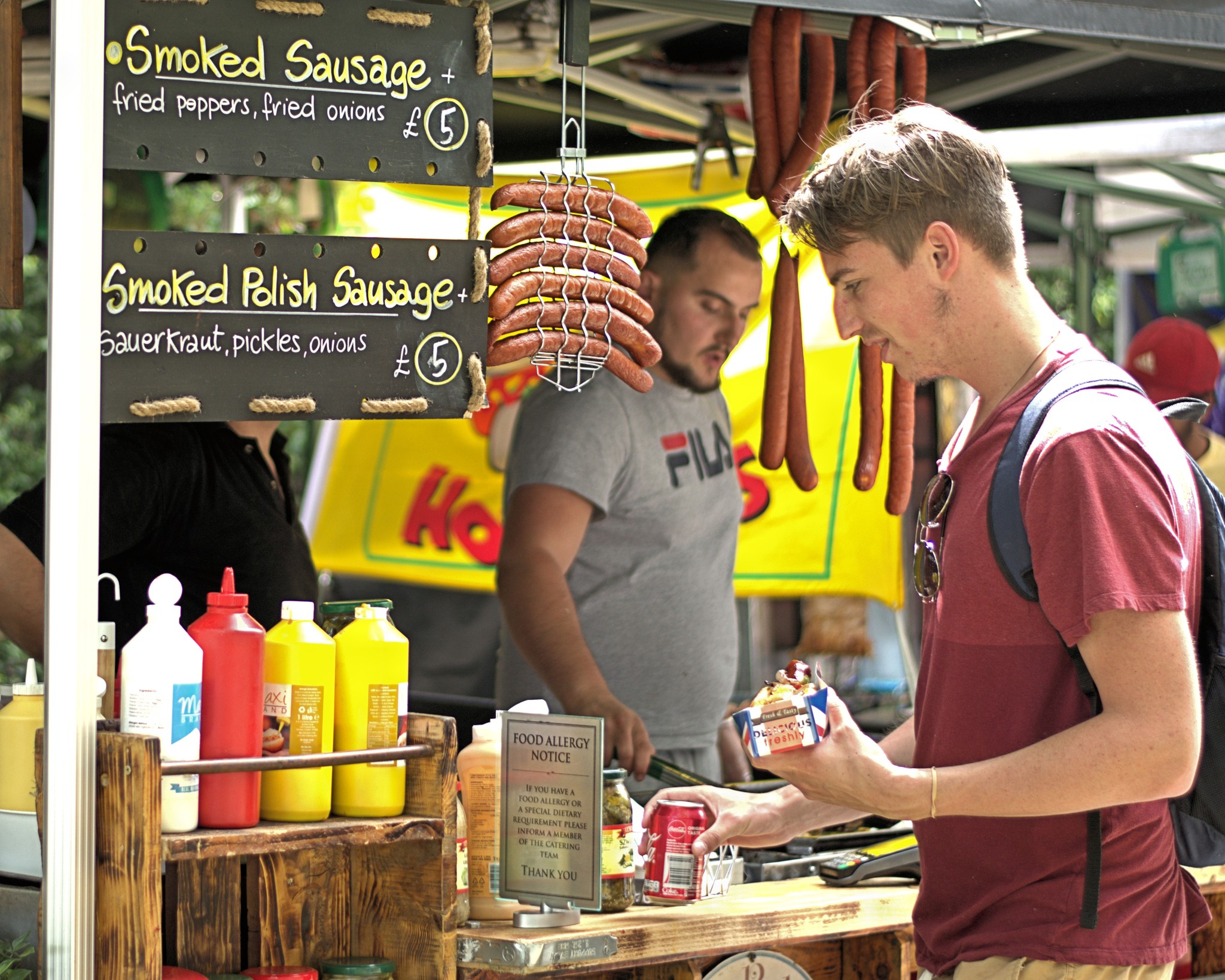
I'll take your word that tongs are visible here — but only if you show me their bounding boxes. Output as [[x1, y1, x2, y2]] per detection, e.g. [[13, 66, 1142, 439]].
[[643, 754, 736, 789]]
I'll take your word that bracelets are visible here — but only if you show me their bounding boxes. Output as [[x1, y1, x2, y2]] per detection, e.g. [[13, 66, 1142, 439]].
[[930, 767, 937, 816]]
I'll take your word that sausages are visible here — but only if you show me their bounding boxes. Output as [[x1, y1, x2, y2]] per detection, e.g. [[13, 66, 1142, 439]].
[[485, 178, 663, 394], [760, 235, 818, 493], [854, 337, 914, 516], [746, 8, 928, 216]]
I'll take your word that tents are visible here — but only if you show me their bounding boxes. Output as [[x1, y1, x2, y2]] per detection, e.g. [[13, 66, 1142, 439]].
[[21, 3, 1224, 205]]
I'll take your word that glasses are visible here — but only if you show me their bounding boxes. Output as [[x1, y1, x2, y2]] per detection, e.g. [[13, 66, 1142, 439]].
[[911, 471, 953, 600]]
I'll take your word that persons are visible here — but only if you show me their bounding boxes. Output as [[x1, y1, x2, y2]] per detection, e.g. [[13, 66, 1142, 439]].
[[1122, 317, 1225, 498], [491, 206, 762, 789], [636, 99, 1214, 979], [1, 420, 322, 718]]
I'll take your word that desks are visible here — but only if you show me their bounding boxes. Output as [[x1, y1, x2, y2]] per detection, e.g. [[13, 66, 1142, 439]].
[[451, 862, 1225, 980]]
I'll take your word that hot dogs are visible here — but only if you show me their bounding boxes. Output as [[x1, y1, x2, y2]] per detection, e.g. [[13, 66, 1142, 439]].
[[748, 656, 817, 707]]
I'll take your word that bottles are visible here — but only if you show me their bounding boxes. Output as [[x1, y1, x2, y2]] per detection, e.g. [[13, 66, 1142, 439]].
[[187, 566, 267, 832], [455, 722, 541, 921], [261, 600, 338, 825], [455, 781, 471, 927], [0, 656, 47, 813], [120, 572, 204, 835], [330, 606, 411, 822]]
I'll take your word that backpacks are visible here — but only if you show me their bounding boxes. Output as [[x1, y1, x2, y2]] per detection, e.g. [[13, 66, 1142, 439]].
[[982, 359, 1224, 867]]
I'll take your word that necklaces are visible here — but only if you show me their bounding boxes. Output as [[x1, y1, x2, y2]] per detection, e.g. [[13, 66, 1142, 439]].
[[993, 318, 1068, 409]]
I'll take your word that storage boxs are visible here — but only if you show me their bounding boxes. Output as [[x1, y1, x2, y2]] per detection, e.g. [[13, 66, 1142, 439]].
[[732, 664, 830, 758]]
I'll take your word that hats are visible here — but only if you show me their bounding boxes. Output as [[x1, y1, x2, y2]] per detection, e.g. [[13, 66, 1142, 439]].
[[1124, 315, 1219, 401]]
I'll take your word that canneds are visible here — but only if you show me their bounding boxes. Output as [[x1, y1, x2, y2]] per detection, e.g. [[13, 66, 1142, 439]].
[[601, 768, 634, 912], [644, 799, 708, 905], [321, 955, 395, 980]]
